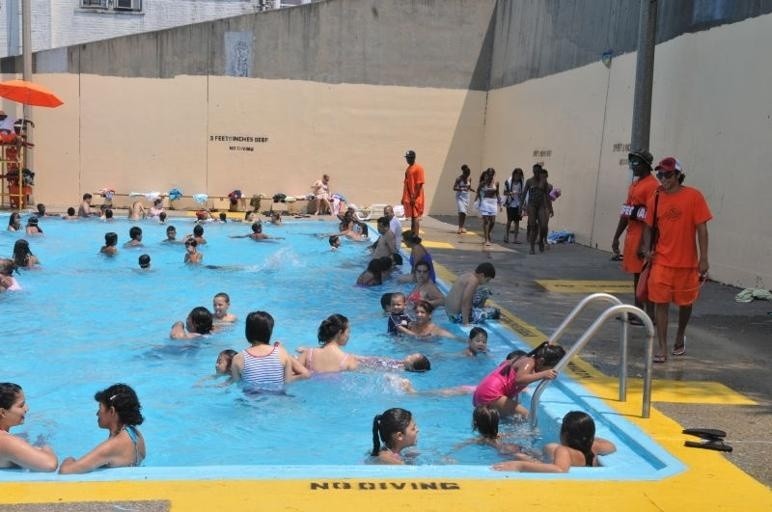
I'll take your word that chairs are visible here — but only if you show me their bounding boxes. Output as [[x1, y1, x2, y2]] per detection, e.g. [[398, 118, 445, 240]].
[[0, 117, 28, 146]]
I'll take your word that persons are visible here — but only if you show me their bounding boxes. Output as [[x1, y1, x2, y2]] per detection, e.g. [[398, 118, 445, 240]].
[[474, 168, 502, 245], [311, 175, 334, 216], [527, 169, 561, 245], [452, 164, 475, 233], [1, 111, 16, 143], [640, 156, 713, 362], [401, 151, 425, 238], [518, 164, 552, 254], [504, 168, 526, 244], [612, 148, 661, 326], [478, 172, 486, 220]]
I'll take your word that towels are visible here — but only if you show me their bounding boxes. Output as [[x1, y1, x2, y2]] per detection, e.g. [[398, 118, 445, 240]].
[[733, 285, 772, 304], [547, 229, 576, 245]]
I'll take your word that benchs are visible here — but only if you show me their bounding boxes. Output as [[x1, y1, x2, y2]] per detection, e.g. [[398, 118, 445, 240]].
[[90, 189, 335, 212]]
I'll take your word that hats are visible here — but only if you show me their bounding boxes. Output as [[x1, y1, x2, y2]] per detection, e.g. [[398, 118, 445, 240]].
[[655, 156, 682, 172], [403, 150, 416, 157], [628, 149, 654, 171]]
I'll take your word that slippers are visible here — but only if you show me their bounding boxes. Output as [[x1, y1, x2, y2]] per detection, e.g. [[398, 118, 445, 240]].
[[670, 335, 688, 356], [630, 318, 656, 326], [652, 352, 667, 364], [682, 428, 728, 438], [616, 315, 636, 321], [684, 438, 732, 452]]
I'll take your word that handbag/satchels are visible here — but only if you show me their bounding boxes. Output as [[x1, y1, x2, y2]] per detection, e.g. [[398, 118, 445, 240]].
[[636, 260, 651, 305]]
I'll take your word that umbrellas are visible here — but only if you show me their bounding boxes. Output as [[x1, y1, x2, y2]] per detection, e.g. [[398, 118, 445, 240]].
[[0, 81, 63, 137]]
